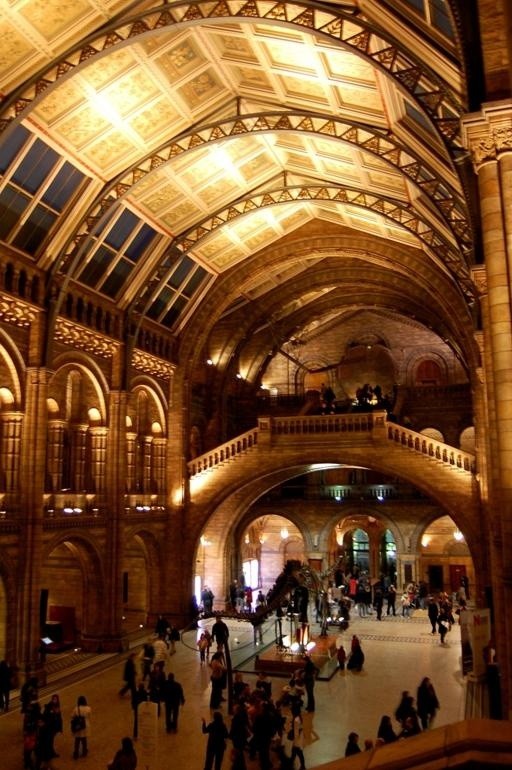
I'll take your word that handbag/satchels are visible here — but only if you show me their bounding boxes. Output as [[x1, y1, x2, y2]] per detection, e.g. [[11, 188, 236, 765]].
[[70, 714, 86, 733]]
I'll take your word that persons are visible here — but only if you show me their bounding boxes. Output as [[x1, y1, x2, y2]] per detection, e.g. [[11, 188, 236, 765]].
[[321, 382, 397, 423], [2, 559, 470, 769]]
